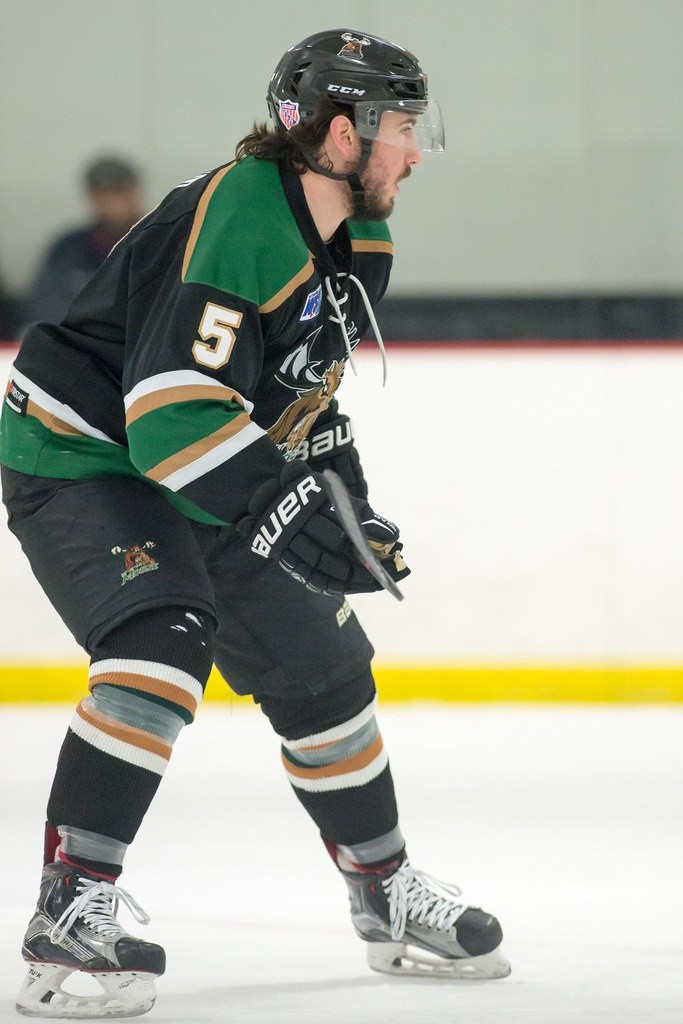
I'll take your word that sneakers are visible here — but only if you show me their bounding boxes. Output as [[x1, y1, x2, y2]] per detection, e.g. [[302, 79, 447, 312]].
[[341, 862, 513, 980], [15, 864, 165, 1018]]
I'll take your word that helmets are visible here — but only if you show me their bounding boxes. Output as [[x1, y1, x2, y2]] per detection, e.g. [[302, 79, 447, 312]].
[[265, 29, 429, 142]]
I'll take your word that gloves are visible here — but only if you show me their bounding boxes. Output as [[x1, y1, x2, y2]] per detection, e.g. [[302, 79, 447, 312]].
[[237, 459, 412, 595], [297, 416, 370, 502]]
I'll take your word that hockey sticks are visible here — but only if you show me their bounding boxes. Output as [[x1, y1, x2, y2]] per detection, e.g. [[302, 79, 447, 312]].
[[323, 467, 404, 603]]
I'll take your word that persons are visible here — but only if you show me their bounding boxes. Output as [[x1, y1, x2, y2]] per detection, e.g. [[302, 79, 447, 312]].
[[25, 159, 146, 337], [0, 29, 510, 1017]]
